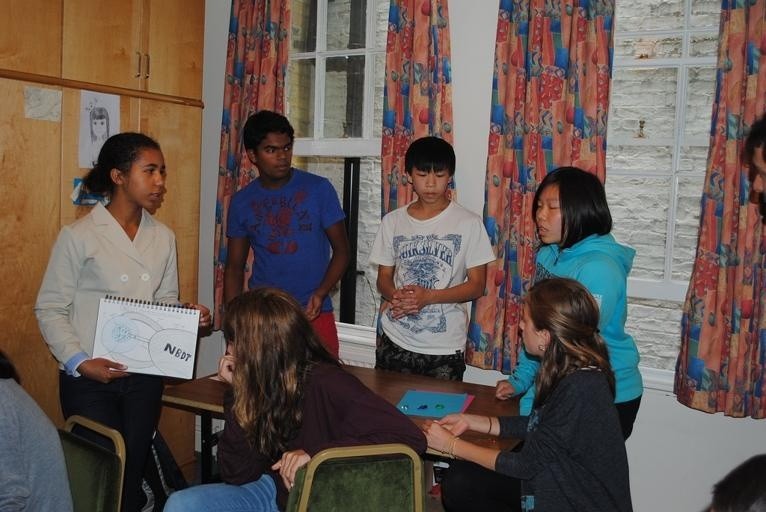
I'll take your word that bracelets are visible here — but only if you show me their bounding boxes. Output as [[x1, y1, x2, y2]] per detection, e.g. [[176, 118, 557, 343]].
[[488, 416, 492, 435], [448, 437, 460, 455]]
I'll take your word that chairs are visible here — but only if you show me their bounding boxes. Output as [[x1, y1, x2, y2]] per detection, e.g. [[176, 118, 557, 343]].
[[59, 414, 126, 512], [286, 444, 423, 512]]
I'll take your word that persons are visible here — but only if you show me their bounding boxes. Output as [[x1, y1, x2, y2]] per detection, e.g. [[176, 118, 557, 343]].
[[220, 105, 353, 360], [368, 134, 497, 382], [89, 106, 110, 142], [0, 347, 74, 512], [421, 276, 634, 512], [704, 454, 766, 512], [157, 285, 431, 512], [494, 165, 645, 442], [33, 130, 213, 512], [746, 112, 766, 202]]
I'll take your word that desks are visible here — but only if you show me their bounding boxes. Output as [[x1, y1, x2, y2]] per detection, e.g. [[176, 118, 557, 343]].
[[162, 358, 527, 511]]
